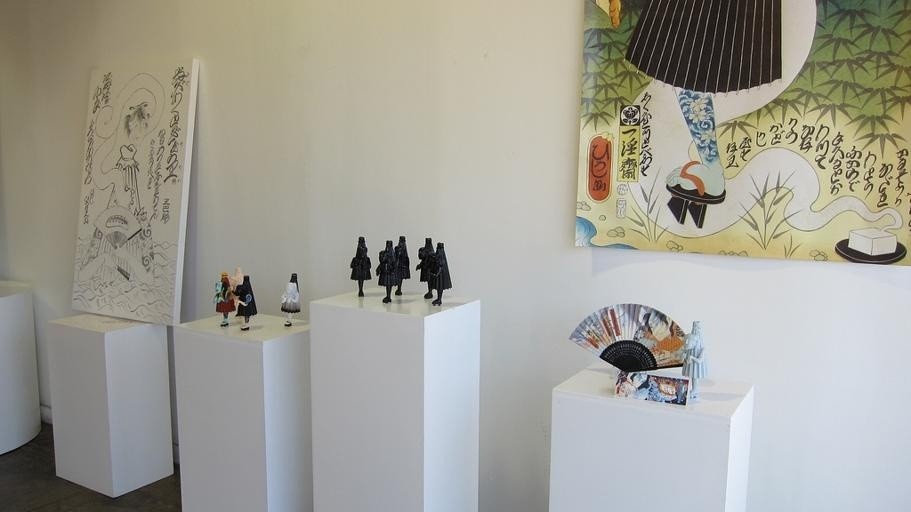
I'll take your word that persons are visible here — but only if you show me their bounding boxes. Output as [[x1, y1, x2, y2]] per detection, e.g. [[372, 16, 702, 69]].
[[395, 236, 410, 294], [212, 273, 236, 327], [350, 237, 371, 297], [376, 240, 395, 303], [234, 274, 257, 329], [280, 273, 301, 326], [682, 321, 708, 400], [415, 238, 436, 299], [429, 243, 453, 305], [231, 267, 243, 290]]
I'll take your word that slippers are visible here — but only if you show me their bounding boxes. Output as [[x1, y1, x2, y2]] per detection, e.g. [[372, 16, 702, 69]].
[[663, 160, 729, 230]]
